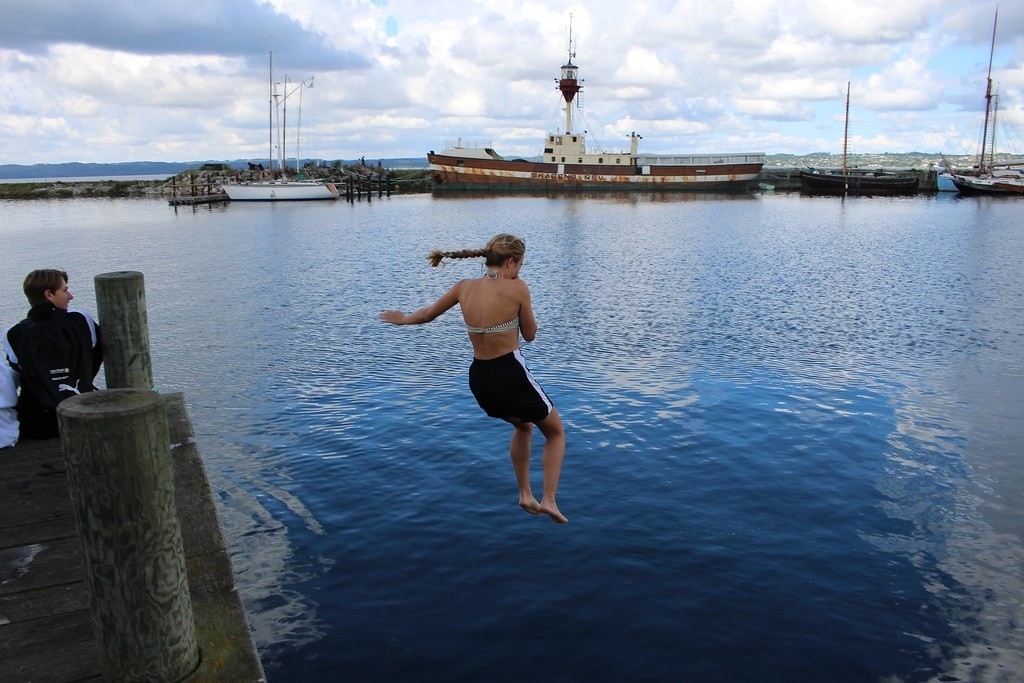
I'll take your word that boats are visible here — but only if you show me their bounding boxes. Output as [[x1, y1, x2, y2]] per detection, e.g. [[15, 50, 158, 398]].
[[171, 8, 1024, 208]]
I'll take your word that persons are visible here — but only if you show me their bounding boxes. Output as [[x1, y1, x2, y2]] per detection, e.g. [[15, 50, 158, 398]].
[[1, 269, 102, 448], [381, 236, 572, 524]]
